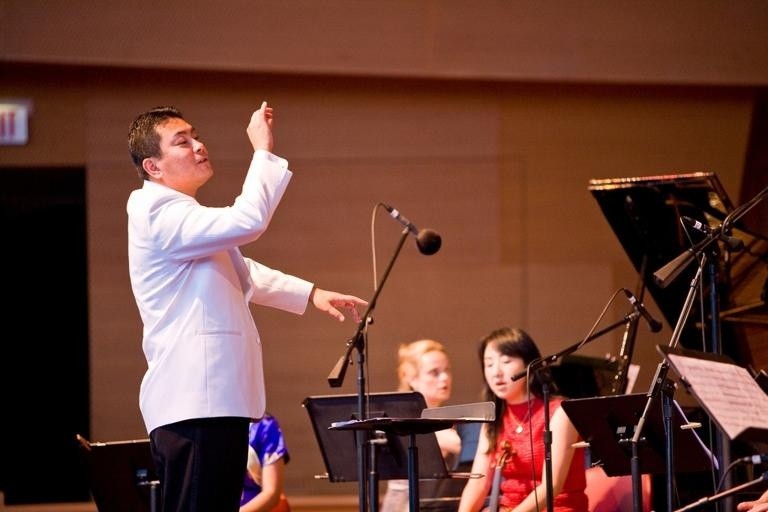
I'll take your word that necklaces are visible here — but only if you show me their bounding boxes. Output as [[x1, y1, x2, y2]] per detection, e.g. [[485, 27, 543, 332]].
[[508, 400, 533, 434]]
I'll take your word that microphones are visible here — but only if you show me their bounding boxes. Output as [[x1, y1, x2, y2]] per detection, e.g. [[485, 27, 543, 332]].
[[623, 289, 663, 333], [386, 205, 442, 256], [683, 216, 744, 253]]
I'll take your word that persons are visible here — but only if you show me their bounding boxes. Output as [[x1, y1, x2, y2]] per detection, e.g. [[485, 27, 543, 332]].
[[455, 324, 591, 512], [372, 334, 462, 512], [237, 412, 293, 512], [123, 97, 373, 512], [734, 488, 768, 511]]
